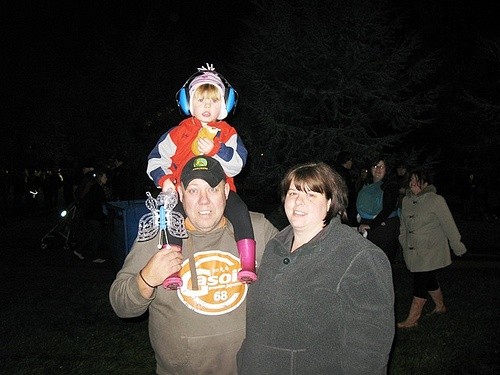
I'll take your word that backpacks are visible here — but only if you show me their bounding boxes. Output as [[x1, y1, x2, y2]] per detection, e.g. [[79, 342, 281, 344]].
[[356, 181, 398, 220]]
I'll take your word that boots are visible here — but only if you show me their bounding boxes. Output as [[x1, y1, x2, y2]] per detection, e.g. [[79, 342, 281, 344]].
[[163, 244, 183, 291], [395, 296, 427, 329], [237, 239, 259, 285], [426, 287, 446, 315]]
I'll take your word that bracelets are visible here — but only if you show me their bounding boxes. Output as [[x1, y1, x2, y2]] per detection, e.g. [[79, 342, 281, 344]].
[[139, 270, 157, 288]]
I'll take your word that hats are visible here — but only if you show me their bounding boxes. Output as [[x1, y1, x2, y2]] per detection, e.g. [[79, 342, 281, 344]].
[[190, 72, 228, 119], [181, 155, 227, 187]]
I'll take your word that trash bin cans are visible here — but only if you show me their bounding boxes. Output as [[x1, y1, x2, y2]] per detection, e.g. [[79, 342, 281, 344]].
[[106, 200, 151, 270]]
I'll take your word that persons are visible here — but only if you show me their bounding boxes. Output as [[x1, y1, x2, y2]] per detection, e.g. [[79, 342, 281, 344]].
[[109, 155, 281, 375], [28, 168, 64, 202], [73, 169, 117, 263], [397, 168, 467, 328], [71, 167, 112, 250], [332, 150, 410, 271], [147, 63, 257, 291], [236, 163, 395, 375]]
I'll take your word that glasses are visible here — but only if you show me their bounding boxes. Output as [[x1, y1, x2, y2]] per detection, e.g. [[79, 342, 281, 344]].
[[372, 165, 384, 169]]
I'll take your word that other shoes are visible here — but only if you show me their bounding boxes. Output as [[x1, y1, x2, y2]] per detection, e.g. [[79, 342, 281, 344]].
[[91, 258, 105, 262], [73, 250, 85, 259]]
